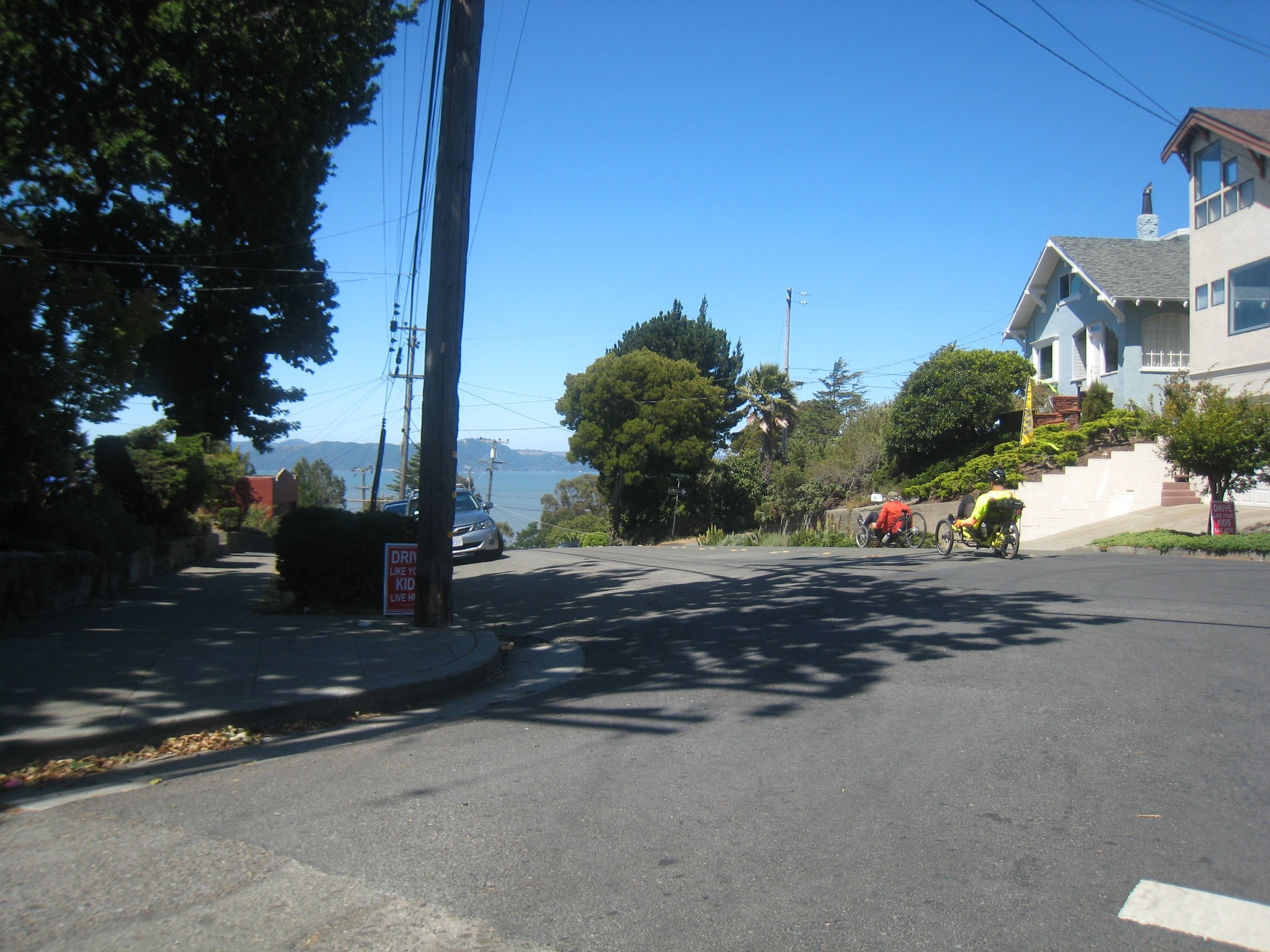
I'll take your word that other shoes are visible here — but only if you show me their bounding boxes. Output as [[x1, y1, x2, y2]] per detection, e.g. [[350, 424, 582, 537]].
[[948, 514, 954, 526], [857, 514, 864, 528]]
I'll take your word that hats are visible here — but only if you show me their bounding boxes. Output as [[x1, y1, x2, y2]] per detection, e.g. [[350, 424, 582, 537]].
[[885, 491, 899, 499], [989, 468, 1006, 484]]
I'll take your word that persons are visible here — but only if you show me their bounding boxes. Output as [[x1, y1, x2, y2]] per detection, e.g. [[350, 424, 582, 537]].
[[857, 491, 913, 535], [948, 469, 1018, 533]]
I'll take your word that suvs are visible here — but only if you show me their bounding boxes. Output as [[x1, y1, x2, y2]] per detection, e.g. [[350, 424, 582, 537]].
[[385, 484, 504, 561]]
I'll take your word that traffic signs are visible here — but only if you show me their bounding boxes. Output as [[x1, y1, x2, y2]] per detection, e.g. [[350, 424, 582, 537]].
[[668, 487, 689, 496]]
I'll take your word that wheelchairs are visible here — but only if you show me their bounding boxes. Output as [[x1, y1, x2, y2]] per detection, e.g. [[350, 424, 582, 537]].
[[856, 510, 927, 548], [935, 499, 1026, 559]]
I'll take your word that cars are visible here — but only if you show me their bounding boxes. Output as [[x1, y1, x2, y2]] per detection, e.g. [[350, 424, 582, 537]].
[[557, 540, 582, 547]]
